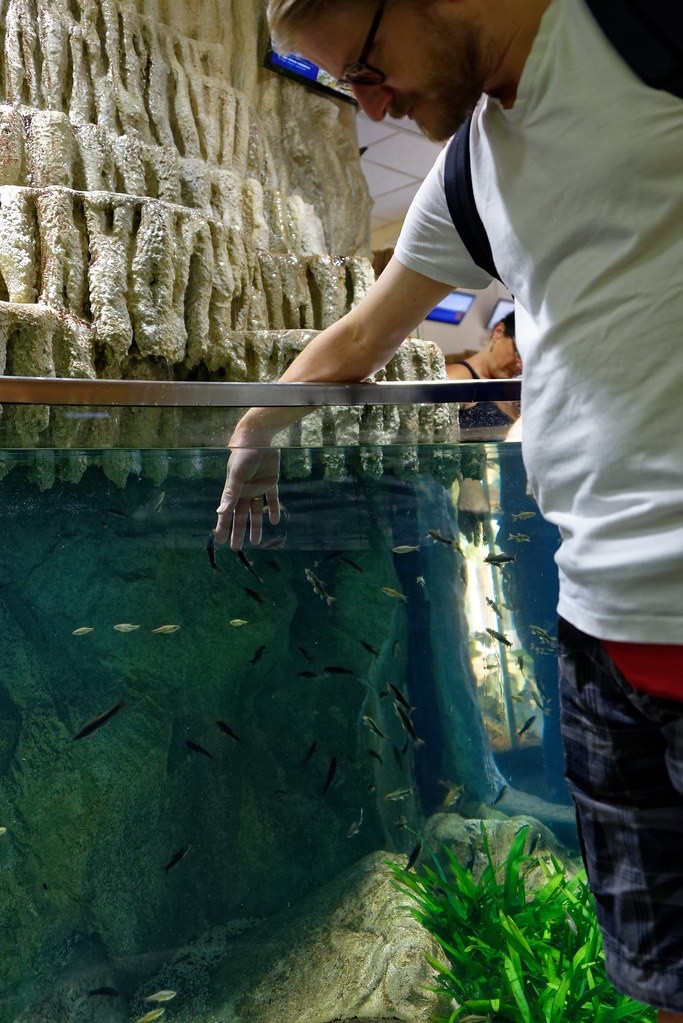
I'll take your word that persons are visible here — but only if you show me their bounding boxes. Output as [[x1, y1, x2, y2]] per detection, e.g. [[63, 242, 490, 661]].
[[444, 310, 522, 443], [457, 443, 582, 860], [212, 0, 683, 1023]]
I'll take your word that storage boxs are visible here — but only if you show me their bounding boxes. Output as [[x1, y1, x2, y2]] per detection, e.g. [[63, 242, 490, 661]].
[[0, 377, 583, 1023]]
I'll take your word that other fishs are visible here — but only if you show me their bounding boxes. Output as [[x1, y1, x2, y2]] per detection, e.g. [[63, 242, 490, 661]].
[[82, 982, 177, 1023], [70, 509, 564, 882]]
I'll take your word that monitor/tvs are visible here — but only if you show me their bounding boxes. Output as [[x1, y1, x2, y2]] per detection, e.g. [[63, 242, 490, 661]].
[[485, 298, 515, 330], [263, 38, 359, 106], [425, 291, 476, 325]]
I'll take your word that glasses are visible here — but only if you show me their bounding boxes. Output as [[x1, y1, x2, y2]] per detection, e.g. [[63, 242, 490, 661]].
[[511, 336, 522, 360], [335, 0, 386, 93]]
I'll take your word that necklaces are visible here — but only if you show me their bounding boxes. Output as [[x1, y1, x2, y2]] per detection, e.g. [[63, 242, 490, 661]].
[[505, 401, 521, 418]]
